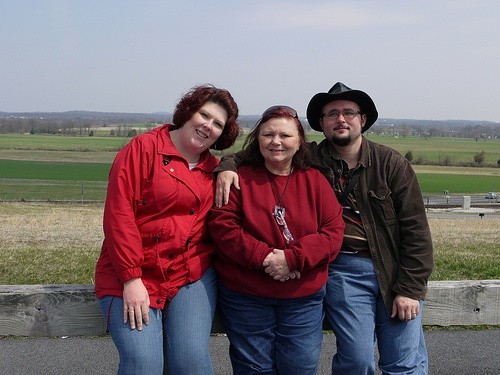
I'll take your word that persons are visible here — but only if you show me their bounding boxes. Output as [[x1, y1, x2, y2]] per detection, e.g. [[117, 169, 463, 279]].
[[206, 105, 345, 375], [95, 86, 239, 375], [212, 82, 433, 375]]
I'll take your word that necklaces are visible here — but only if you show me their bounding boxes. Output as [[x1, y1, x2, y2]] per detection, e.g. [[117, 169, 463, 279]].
[[265, 166, 294, 205]]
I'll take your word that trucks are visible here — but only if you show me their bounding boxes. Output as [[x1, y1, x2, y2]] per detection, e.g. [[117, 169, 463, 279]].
[[485, 192, 496, 199]]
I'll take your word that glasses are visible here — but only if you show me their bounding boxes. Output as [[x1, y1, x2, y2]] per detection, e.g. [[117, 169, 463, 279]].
[[262, 105, 299, 123], [324, 110, 361, 119], [273, 212, 293, 244]]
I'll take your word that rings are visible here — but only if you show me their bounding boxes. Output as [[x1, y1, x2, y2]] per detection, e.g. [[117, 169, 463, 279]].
[[411, 313, 417, 315]]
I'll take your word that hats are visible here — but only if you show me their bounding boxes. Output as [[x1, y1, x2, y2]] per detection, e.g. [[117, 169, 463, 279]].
[[307, 81, 378, 133]]
